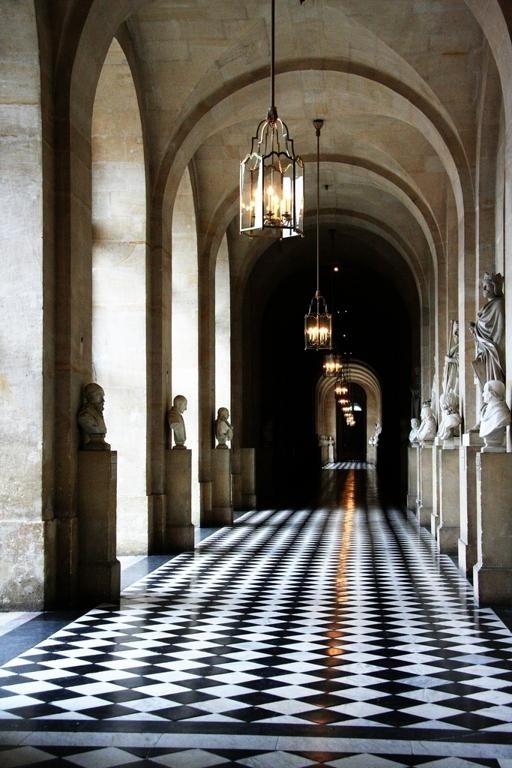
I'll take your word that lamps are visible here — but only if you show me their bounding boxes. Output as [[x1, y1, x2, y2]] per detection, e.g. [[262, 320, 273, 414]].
[[239, 0, 308, 240], [322, 236, 357, 427], [304, 118, 332, 351]]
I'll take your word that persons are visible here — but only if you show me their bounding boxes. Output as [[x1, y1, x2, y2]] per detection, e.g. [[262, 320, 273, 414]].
[[442, 320, 460, 394], [413, 405, 437, 441], [465, 271, 505, 433], [477, 379, 511, 447], [437, 393, 462, 442], [168, 396, 188, 447], [215, 406, 234, 447], [76, 383, 107, 436], [408, 417, 420, 442]]
